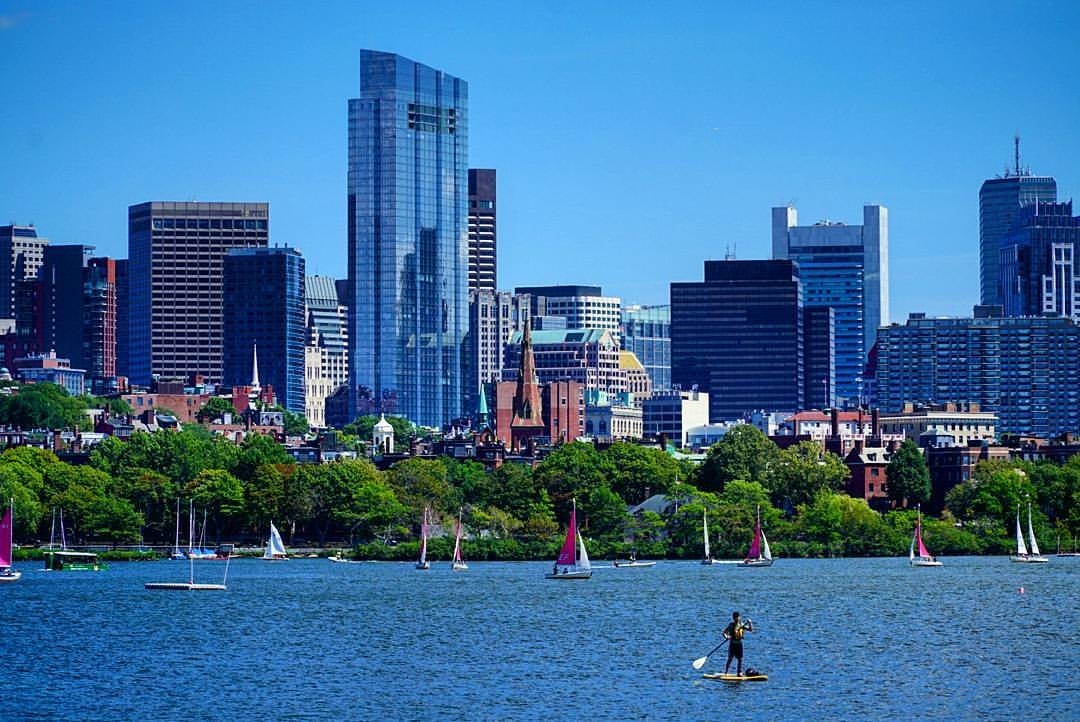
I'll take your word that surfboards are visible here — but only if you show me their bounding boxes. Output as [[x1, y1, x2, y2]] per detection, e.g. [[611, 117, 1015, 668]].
[[702, 672, 769, 682]]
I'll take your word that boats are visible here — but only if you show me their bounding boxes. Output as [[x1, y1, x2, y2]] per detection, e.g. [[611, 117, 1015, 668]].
[[328, 552, 349, 563], [614, 553, 657, 567], [215, 544, 242, 560], [1056, 552, 1080, 557], [38, 508, 100, 571]]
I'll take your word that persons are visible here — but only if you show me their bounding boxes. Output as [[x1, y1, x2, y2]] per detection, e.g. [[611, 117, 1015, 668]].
[[563, 567, 568, 574], [933, 555, 936, 561], [7, 569, 11, 575], [722, 611, 753, 677], [928, 554, 932, 561], [336, 552, 345, 559], [553, 565, 558, 575]]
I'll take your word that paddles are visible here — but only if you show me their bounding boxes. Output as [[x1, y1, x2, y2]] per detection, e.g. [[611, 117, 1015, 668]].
[[691, 619, 752, 670]]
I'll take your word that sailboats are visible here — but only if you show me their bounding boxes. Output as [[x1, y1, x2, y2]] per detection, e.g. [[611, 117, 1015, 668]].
[[258, 520, 289, 560], [701, 508, 714, 565], [543, 497, 594, 579], [1007, 502, 1049, 562], [417, 506, 429, 570], [170, 497, 218, 560], [910, 502, 943, 566], [451, 508, 468, 570], [0, 498, 22, 581], [737, 504, 780, 567]]
